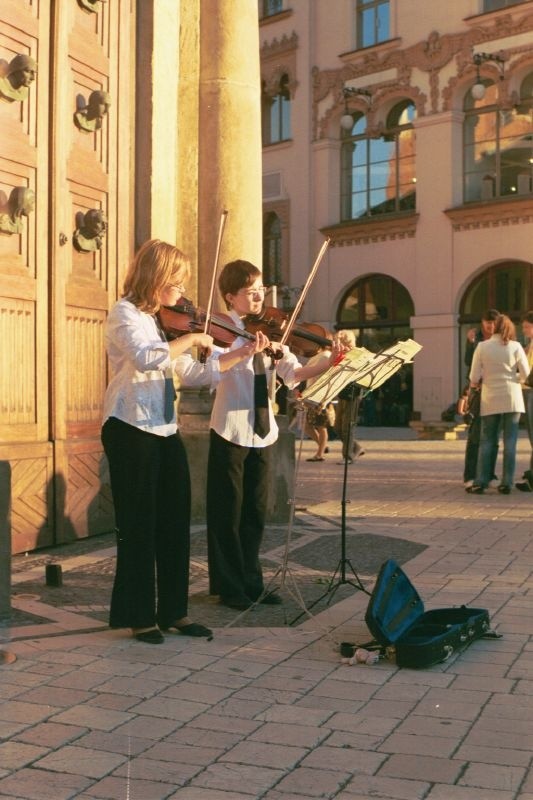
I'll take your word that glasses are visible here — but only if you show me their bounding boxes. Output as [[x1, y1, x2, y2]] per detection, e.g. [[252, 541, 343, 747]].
[[240, 286, 267, 296]]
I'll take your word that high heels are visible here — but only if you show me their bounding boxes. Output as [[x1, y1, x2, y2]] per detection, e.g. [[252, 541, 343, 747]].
[[158, 621, 212, 637], [133, 629, 165, 643]]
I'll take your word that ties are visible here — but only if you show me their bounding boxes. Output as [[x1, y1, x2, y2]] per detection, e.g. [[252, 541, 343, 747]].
[[252, 352, 270, 439], [150, 315, 175, 423]]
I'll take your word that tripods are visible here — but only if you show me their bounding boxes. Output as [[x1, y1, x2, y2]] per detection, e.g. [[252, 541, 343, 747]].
[[289, 352, 410, 625], [225, 350, 399, 628]]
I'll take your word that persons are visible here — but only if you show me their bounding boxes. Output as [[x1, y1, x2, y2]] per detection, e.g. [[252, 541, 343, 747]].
[[200, 258, 351, 610], [293, 322, 366, 466], [464, 314, 531, 494], [520, 310, 533, 492], [461, 309, 504, 489], [100, 239, 271, 643]]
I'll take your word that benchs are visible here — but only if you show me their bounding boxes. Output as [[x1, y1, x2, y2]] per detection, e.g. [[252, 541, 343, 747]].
[[409, 420, 471, 440]]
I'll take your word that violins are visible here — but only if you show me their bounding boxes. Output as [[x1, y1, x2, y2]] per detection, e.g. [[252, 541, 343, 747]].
[[242, 306, 352, 358], [154, 295, 284, 360]]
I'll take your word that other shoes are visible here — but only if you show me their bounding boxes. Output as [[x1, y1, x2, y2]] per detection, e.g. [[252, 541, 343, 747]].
[[336, 459, 354, 464], [516, 481, 533, 492], [497, 484, 511, 494], [465, 485, 484, 494], [246, 588, 283, 603], [523, 470, 533, 481], [323, 448, 328, 453], [218, 594, 255, 608], [352, 448, 364, 460], [307, 457, 325, 461]]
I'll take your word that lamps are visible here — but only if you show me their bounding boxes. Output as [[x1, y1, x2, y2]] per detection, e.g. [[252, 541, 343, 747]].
[[470, 52, 505, 99], [339, 87, 372, 130]]
